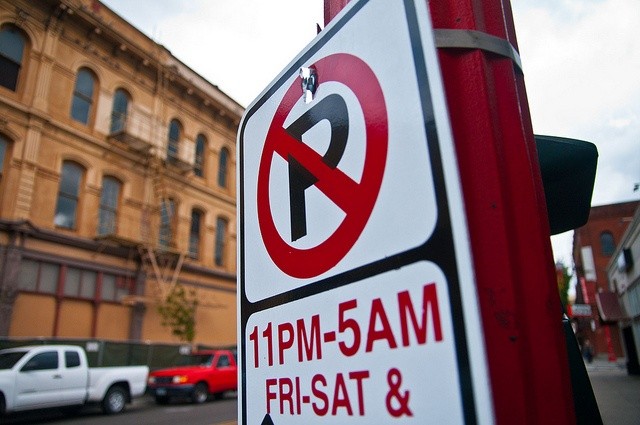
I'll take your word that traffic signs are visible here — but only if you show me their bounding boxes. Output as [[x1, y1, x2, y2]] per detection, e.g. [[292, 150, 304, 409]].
[[232, 0, 495, 425]]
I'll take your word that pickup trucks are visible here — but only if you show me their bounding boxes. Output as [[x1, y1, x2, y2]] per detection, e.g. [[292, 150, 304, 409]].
[[0, 345, 149, 423], [149, 350, 237, 404]]
